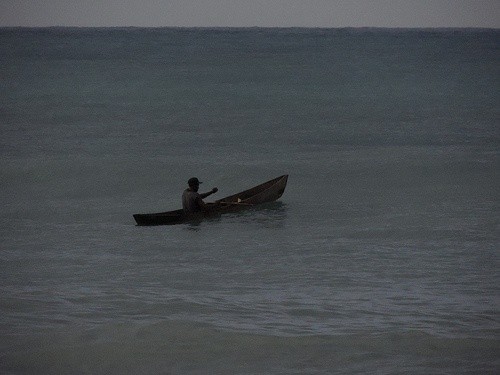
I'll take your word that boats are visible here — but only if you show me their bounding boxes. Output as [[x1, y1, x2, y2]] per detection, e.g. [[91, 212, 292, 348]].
[[132, 175, 289, 225]]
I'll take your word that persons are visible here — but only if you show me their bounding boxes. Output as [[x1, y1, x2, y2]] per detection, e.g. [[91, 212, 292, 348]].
[[182, 177, 218, 213]]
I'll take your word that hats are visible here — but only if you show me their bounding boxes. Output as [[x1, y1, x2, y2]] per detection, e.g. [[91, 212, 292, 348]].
[[187, 178, 203, 184]]
